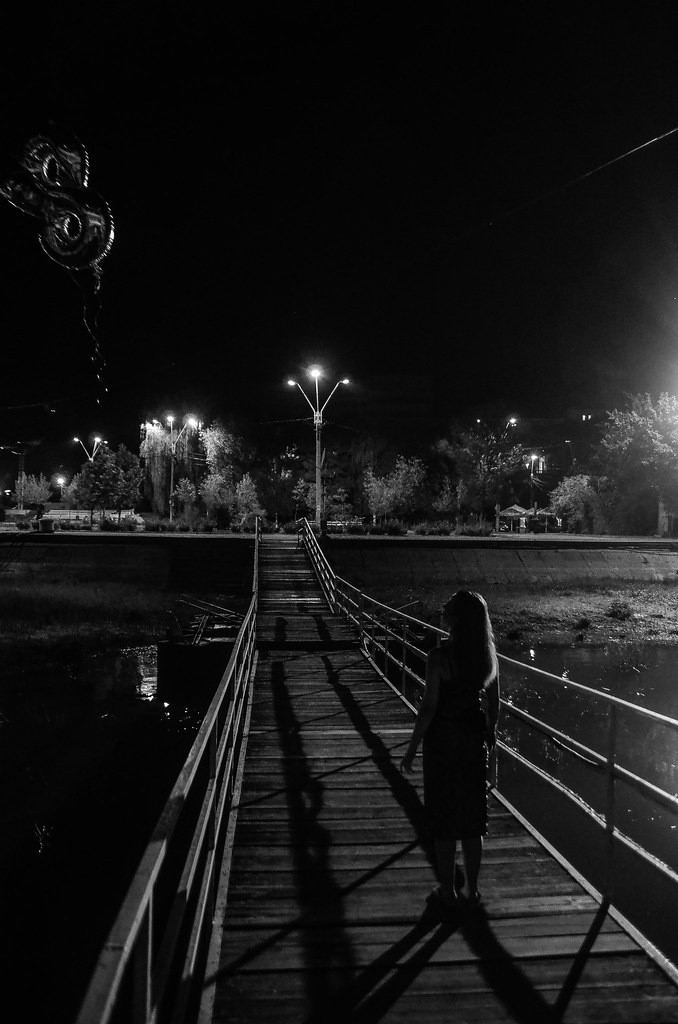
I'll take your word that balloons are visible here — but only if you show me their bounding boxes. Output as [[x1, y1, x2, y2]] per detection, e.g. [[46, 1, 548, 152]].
[[0, 130, 114, 270]]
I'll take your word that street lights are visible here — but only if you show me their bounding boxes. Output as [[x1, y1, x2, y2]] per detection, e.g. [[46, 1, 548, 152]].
[[476, 417, 517, 532], [148, 413, 199, 524], [288, 364, 349, 522], [73, 436, 108, 520]]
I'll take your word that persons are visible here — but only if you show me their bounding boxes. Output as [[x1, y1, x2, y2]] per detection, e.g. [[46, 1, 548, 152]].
[[400, 590, 500, 906]]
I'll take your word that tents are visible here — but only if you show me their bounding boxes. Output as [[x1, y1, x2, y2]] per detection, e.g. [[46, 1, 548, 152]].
[[497, 505, 557, 532]]
